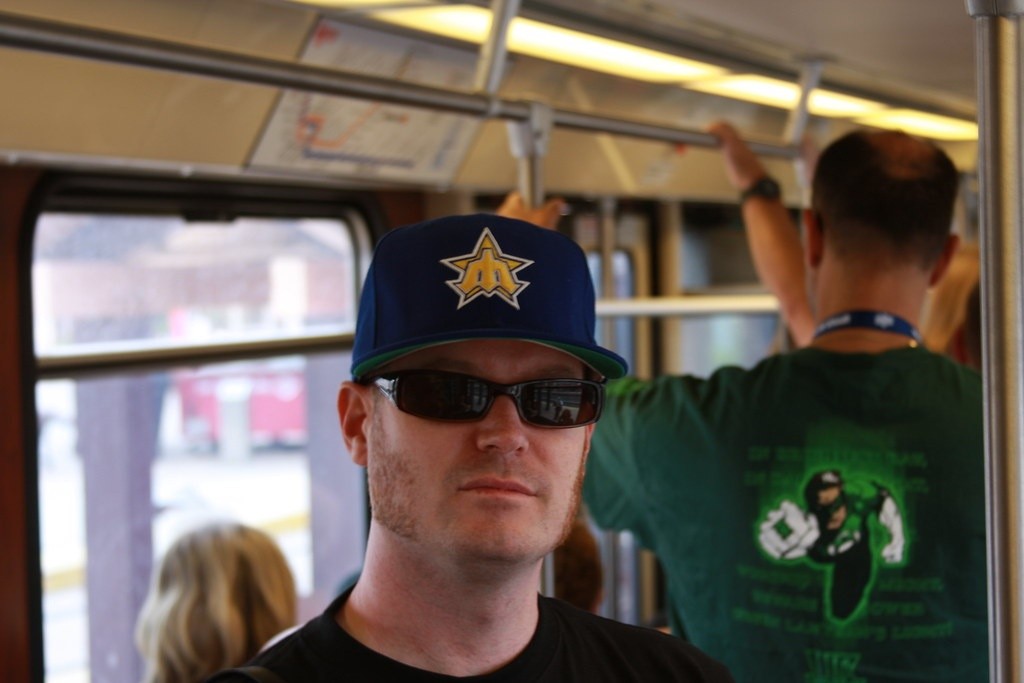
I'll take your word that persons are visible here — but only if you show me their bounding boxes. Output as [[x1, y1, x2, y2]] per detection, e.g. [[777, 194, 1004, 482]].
[[708, 111, 982, 367], [496, 125, 988, 683], [554, 522, 605, 613], [132, 521, 299, 683], [199, 214, 734, 683]]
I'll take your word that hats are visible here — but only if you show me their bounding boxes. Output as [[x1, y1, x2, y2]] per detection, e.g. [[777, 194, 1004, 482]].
[[351, 211, 630, 387]]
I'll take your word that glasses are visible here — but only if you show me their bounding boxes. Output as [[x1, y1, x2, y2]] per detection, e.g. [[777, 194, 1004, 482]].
[[362, 368, 607, 429]]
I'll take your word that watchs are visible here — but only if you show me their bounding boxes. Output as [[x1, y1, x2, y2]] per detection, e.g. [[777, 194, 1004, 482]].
[[738, 175, 781, 212]]
